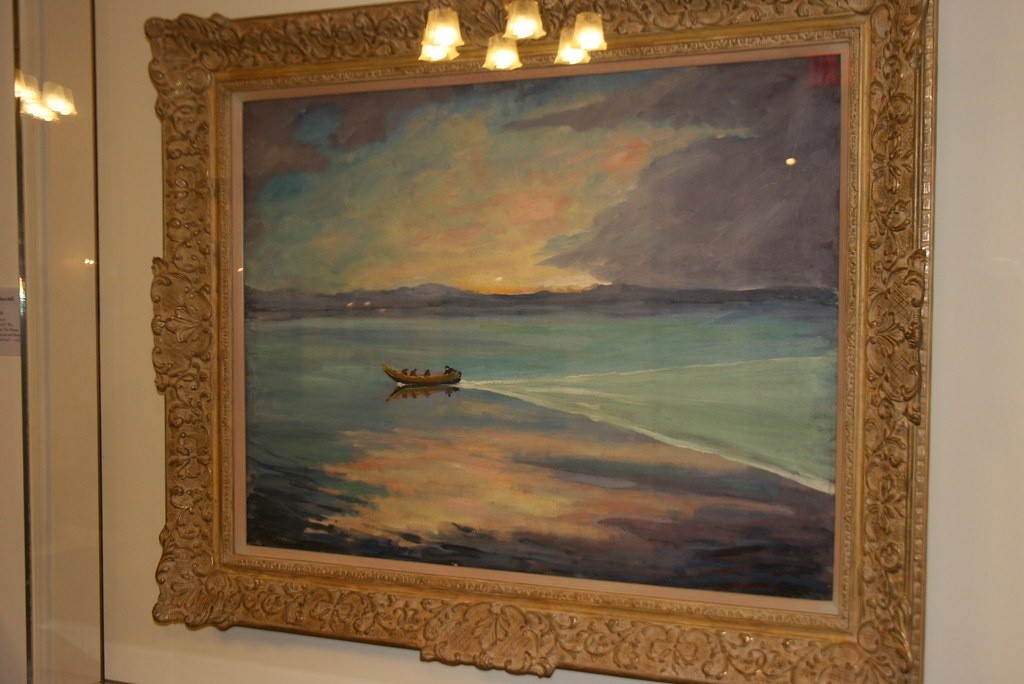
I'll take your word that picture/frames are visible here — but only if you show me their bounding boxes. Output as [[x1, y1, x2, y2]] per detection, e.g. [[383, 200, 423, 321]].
[[143, 0, 938, 684]]
